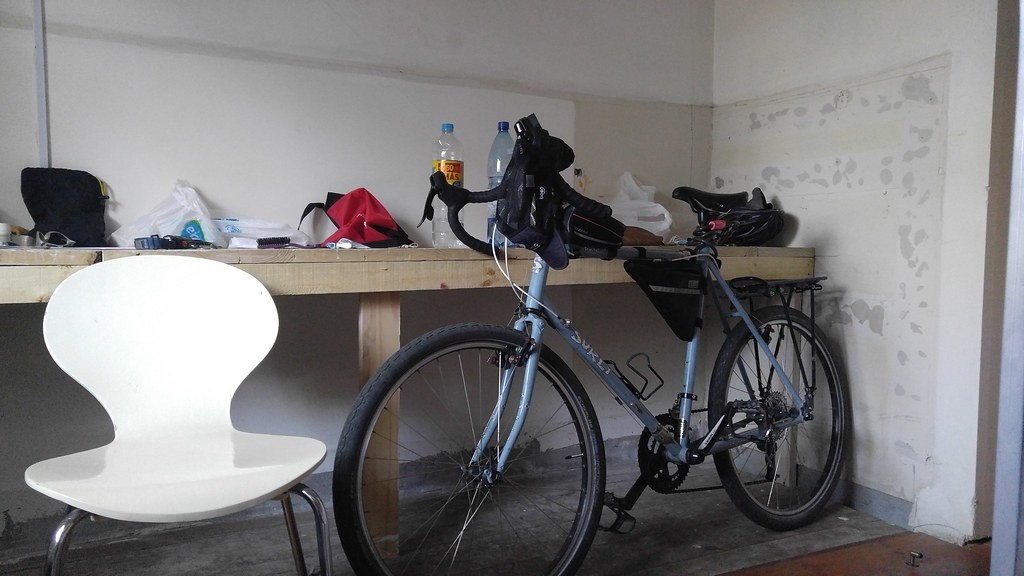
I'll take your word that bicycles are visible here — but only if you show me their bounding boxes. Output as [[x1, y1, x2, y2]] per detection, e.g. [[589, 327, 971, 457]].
[[331, 112, 852, 576]]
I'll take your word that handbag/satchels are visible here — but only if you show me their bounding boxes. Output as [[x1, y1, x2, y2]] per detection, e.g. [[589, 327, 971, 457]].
[[605, 171, 673, 242], [106, 179, 310, 250], [298, 187, 414, 249], [20, 167, 110, 246]]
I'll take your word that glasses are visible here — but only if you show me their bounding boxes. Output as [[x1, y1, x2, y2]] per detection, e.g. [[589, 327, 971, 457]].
[[36, 230, 76, 249]]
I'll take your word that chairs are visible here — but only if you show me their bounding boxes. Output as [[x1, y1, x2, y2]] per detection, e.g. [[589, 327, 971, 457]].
[[22, 255, 335, 576]]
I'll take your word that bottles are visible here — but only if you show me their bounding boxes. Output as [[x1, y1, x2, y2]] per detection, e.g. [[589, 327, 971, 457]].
[[487, 121, 514, 247], [433, 124, 464, 248]]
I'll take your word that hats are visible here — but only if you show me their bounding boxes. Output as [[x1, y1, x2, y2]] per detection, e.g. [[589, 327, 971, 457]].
[[497, 129, 569, 270]]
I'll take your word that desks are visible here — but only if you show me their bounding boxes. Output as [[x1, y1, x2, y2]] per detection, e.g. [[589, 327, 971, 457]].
[[0, 245, 815, 562]]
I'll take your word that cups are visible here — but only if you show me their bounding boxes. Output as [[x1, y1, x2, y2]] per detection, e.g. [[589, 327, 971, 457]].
[[0, 223, 12, 243]]
